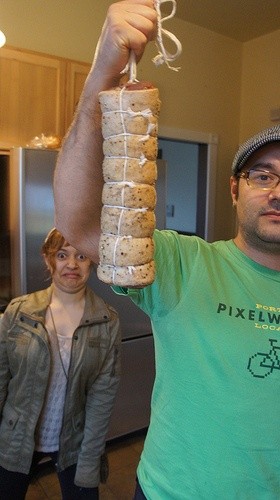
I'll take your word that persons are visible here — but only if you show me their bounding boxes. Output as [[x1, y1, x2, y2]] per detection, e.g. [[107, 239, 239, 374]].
[[53, 0, 280, 500], [0, 228, 122, 500]]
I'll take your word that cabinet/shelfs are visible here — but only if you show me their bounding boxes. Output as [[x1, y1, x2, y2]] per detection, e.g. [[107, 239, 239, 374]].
[[0, 44, 92, 308]]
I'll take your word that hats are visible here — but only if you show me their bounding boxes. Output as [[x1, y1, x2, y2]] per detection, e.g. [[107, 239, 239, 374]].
[[231, 124, 280, 177]]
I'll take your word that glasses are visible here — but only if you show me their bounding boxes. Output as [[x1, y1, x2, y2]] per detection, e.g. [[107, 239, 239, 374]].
[[235, 168, 280, 191]]
